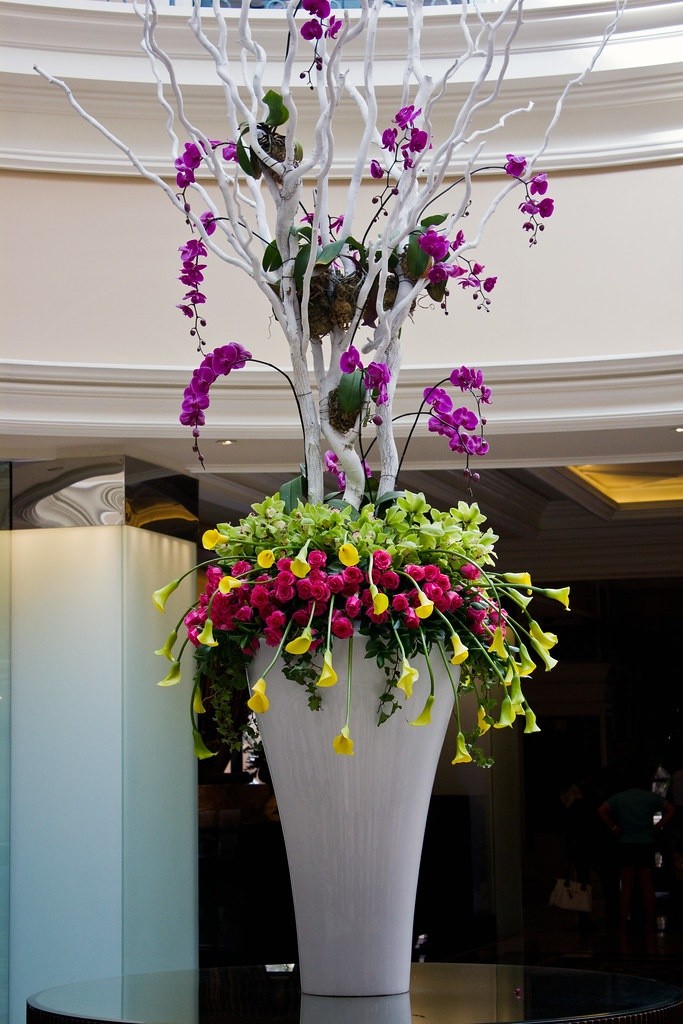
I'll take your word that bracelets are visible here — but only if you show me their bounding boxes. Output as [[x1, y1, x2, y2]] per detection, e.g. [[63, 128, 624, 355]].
[[608, 824, 617, 831]]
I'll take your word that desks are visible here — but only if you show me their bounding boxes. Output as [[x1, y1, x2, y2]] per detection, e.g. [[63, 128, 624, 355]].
[[26, 962, 683, 1024]]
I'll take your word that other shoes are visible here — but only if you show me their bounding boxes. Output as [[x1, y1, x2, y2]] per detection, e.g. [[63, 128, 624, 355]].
[[647, 944, 671, 955], [620, 943, 632, 953]]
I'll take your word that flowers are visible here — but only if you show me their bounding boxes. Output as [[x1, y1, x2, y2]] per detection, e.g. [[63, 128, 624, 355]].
[[29, 0, 621, 768]]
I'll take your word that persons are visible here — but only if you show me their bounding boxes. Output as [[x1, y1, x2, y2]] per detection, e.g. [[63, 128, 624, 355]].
[[558, 769, 682, 963]]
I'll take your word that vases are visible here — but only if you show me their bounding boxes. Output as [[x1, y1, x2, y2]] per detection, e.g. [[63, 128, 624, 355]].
[[241, 635, 462, 999]]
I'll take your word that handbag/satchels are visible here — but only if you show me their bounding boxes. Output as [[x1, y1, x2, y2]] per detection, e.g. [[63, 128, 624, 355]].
[[548, 878, 592, 912]]
